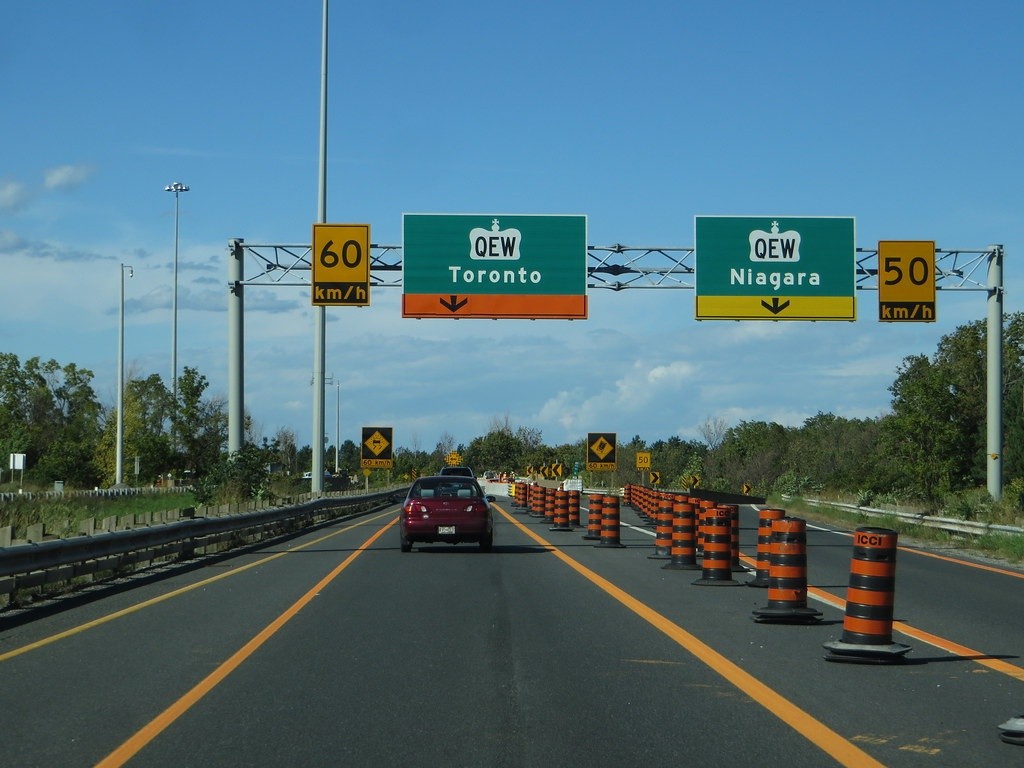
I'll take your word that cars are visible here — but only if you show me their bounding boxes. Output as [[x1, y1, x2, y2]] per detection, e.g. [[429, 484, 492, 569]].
[[437, 467, 475, 479], [400, 474, 497, 548]]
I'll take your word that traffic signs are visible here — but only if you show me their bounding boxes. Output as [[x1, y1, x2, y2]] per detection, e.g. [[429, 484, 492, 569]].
[[404, 213, 589, 321], [697, 215, 856, 322]]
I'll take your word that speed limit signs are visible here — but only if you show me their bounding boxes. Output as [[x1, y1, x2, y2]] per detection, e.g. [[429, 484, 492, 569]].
[[637, 453, 652, 471], [878, 239, 937, 325], [312, 223, 373, 307]]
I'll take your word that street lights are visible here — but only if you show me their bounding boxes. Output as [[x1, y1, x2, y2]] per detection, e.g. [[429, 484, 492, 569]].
[[163, 182, 191, 433], [116, 263, 134, 487]]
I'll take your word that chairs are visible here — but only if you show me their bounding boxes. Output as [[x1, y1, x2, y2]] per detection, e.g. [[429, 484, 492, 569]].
[[421, 489, 434, 497], [457, 489, 470, 496]]
